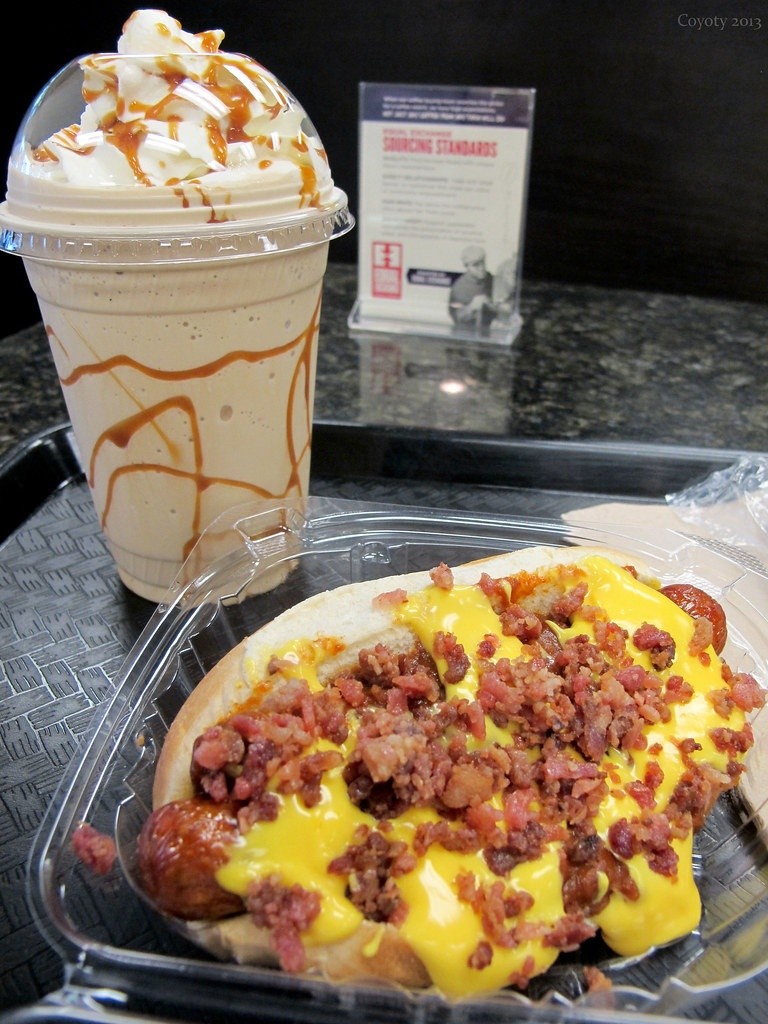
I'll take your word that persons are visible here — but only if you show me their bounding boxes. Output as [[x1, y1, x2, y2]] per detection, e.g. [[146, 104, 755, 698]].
[[447, 244, 496, 335]]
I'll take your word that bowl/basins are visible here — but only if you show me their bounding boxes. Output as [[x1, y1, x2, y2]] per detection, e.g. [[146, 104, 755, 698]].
[[23, 496, 768, 1023]]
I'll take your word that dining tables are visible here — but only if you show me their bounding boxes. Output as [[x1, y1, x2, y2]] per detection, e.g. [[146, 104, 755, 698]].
[[0, 246, 768, 1024]]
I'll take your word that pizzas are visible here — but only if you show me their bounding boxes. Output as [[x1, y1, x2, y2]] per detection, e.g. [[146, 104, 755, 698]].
[[136, 545, 767, 1002]]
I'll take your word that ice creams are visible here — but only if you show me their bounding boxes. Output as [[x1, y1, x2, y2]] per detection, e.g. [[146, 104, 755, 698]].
[[1, 6, 354, 610]]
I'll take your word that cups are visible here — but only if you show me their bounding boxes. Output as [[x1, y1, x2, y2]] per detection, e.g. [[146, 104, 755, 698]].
[[0, 48, 352, 608]]
